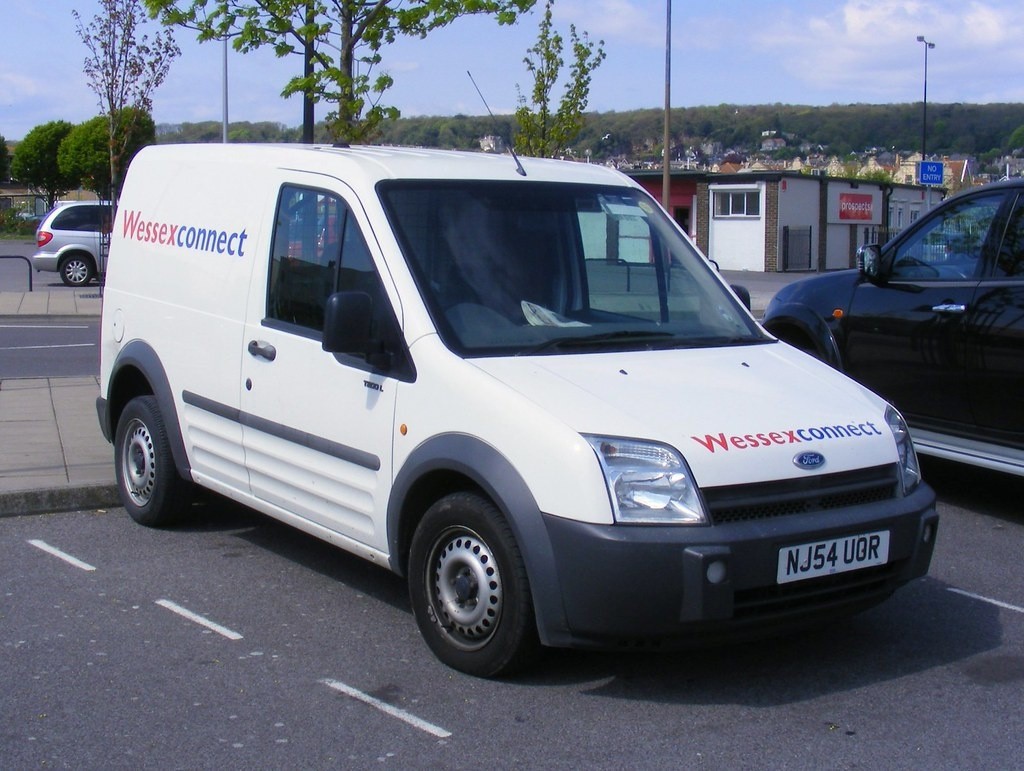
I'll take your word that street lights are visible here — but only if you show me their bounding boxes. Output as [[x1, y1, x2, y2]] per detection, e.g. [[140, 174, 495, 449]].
[[916, 34, 937, 199]]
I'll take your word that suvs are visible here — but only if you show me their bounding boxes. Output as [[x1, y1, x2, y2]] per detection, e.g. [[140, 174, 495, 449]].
[[760, 175, 1023, 483]]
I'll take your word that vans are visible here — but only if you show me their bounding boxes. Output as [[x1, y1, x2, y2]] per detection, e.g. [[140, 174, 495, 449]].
[[93, 139, 936, 685]]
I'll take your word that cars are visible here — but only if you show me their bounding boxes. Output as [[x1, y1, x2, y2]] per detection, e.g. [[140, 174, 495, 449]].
[[30, 200, 121, 287]]
